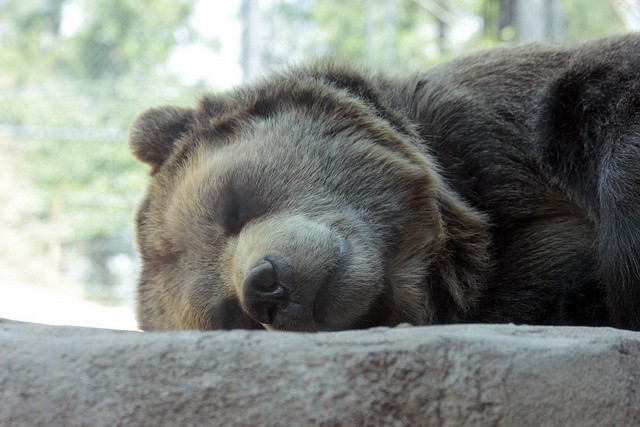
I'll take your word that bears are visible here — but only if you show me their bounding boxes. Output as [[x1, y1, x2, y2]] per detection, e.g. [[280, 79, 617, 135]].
[[127, 30, 639, 334]]
